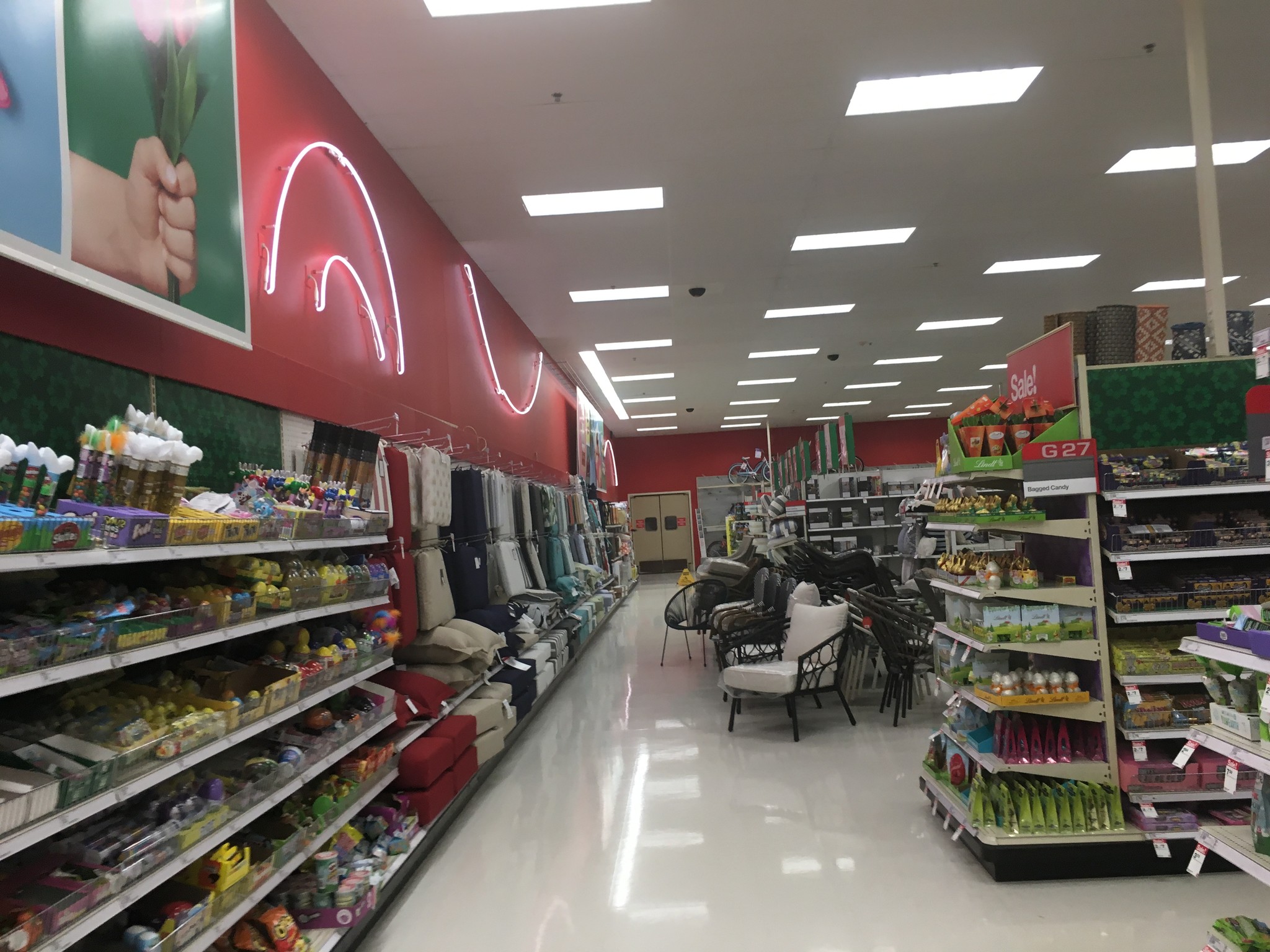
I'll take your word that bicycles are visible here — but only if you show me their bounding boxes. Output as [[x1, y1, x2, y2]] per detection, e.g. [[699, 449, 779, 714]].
[[811, 451, 864, 476], [706, 534, 728, 557], [754, 447, 790, 482], [728, 448, 780, 484]]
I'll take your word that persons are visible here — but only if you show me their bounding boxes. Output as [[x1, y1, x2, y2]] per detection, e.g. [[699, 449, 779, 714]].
[[70, 134, 198, 298]]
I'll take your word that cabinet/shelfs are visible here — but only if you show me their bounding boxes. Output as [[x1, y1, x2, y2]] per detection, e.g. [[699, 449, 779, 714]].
[[744, 323, 1270, 952], [0, 476, 640, 952]]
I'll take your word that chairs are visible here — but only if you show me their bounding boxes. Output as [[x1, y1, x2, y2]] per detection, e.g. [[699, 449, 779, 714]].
[[659, 536, 936, 743]]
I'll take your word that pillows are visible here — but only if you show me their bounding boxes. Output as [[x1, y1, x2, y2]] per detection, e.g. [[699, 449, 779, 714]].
[[378, 449, 627, 826]]
